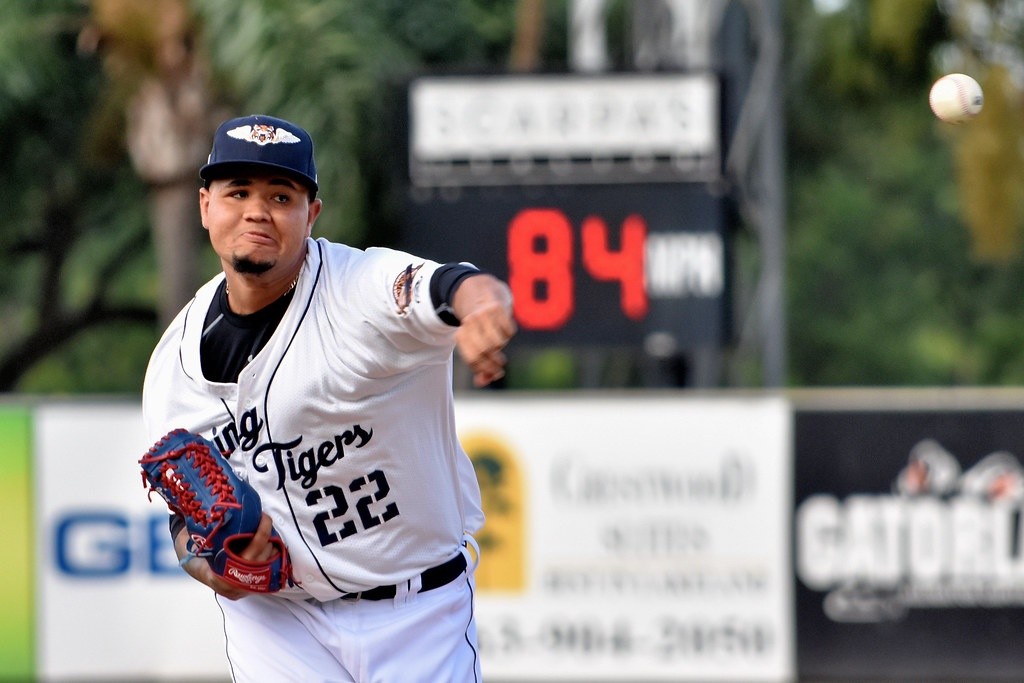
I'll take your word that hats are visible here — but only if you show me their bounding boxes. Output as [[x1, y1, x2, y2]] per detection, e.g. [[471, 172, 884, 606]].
[[198, 113, 319, 203]]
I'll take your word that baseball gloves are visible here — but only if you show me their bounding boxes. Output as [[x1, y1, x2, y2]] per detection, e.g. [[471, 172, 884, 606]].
[[134, 426, 307, 597]]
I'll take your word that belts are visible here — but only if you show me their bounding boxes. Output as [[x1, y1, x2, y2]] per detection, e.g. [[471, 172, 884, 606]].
[[341, 552, 467, 600]]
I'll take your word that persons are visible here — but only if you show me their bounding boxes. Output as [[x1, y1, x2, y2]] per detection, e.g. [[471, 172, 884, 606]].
[[138, 114, 517, 683]]
